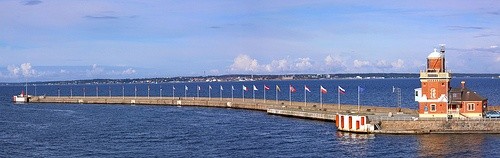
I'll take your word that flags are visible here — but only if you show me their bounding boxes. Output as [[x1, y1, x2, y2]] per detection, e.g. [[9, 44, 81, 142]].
[[276, 85, 281, 92], [305, 85, 311, 93], [360, 88, 365, 95], [233, 87, 236, 90], [243, 85, 248, 91], [265, 86, 269, 90], [339, 87, 346, 95], [254, 85, 258, 90], [173, 85, 223, 90], [321, 86, 327, 94], [290, 85, 296, 92]]
